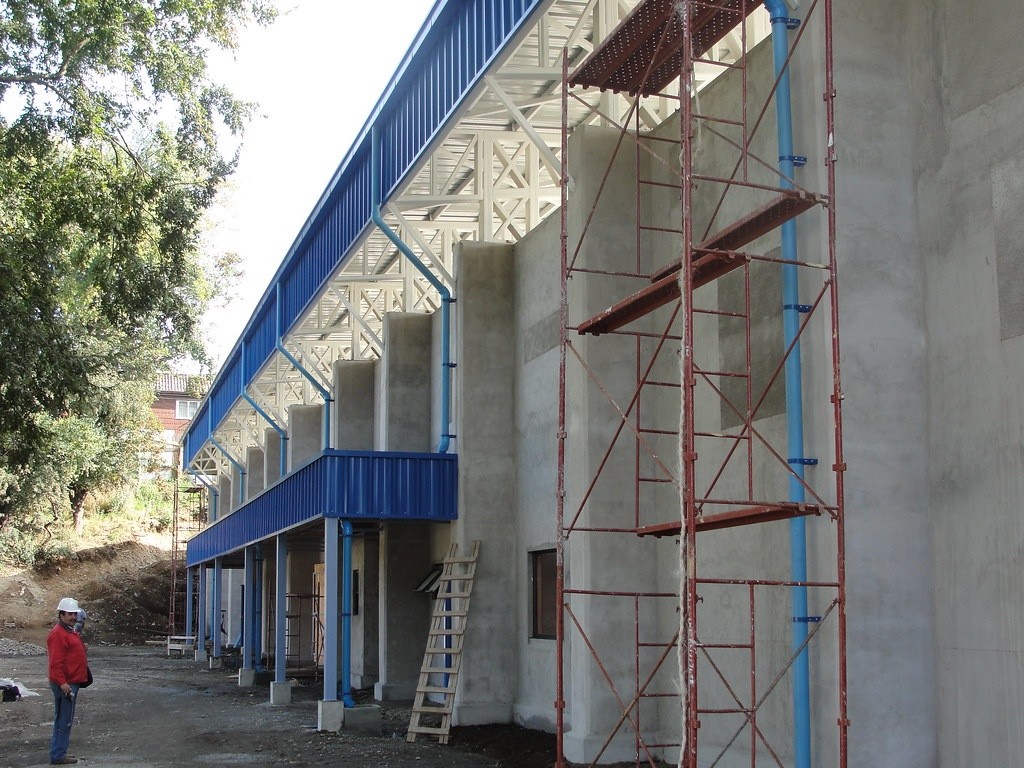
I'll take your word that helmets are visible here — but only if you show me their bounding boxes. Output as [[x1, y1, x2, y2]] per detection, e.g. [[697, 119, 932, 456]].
[[57, 598, 81, 612]]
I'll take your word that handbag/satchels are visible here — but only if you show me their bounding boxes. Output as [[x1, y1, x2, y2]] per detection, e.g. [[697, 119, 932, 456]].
[[79, 665, 93, 688], [0, 685, 21, 702]]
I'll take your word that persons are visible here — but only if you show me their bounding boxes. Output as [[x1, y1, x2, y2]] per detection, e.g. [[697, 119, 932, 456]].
[[47, 597, 94, 765]]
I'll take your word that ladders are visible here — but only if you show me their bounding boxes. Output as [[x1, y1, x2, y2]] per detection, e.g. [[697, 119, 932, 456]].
[[406, 541, 480, 745]]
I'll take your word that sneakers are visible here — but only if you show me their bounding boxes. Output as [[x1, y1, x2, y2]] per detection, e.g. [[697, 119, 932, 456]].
[[51, 755, 78, 765]]
